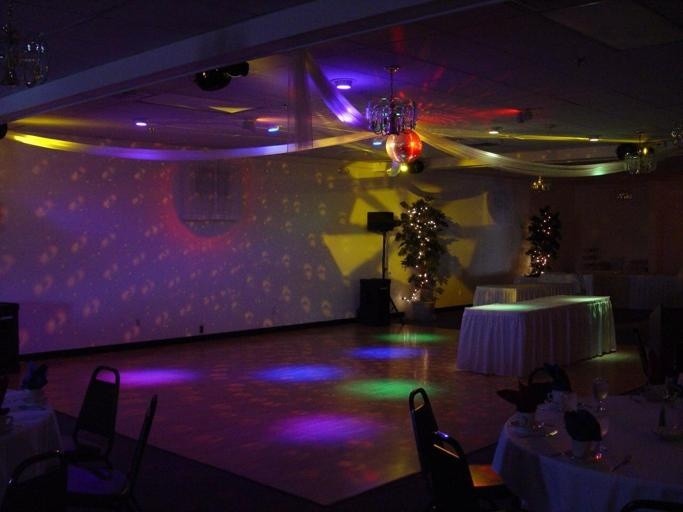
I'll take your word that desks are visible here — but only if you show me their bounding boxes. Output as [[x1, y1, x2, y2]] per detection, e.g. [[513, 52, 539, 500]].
[[457, 271, 683, 381]]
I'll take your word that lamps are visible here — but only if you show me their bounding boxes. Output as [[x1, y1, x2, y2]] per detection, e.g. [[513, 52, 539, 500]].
[[0, 0, 51, 88], [365, 65, 416, 137], [625, 129, 656, 175]]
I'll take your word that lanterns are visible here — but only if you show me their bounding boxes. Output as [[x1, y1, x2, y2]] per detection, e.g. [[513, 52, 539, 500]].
[[385, 128, 424, 165]]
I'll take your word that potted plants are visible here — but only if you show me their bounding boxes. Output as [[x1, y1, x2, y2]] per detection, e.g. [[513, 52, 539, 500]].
[[395, 201, 448, 323]]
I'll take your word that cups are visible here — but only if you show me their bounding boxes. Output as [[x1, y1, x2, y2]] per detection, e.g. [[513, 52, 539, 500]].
[[23, 384, 48, 402], [515, 409, 536, 427], [546, 390, 569, 404], [591, 375, 609, 417]]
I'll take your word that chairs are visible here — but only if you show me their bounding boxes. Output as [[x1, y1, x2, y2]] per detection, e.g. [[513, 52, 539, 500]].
[[622, 499, 683, 512], [0, 366, 158, 512], [405, 363, 572, 512]]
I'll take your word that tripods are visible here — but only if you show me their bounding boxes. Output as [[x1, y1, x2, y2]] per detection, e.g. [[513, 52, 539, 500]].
[[382, 236, 405, 326]]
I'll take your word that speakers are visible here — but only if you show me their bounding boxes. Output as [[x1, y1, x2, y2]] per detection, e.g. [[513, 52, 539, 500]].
[[365, 211, 394, 232], [360, 277, 391, 328]]
